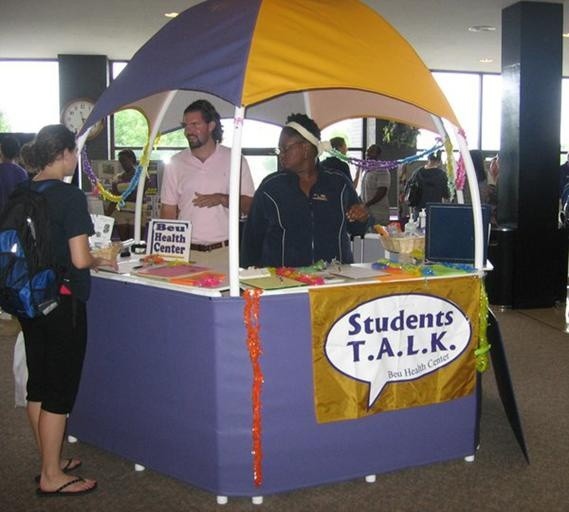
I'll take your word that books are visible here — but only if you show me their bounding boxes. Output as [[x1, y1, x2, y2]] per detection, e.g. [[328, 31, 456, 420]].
[[136, 262, 210, 281]]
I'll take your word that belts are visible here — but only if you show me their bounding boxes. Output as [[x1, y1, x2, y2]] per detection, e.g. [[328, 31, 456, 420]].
[[191, 240, 229, 251]]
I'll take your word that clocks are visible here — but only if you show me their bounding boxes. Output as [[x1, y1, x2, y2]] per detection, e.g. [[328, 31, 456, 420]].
[[60, 98, 105, 141]]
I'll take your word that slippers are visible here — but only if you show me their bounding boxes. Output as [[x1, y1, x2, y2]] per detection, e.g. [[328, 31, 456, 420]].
[[37, 475, 98, 498], [33, 456, 79, 482]]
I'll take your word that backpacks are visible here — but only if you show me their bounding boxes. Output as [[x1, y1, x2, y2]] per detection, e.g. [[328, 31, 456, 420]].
[[0, 180, 74, 321]]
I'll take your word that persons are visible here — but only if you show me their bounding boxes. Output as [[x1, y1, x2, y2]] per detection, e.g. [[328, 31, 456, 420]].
[[238, 113, 370, 268], [107, 147, 152, 254], [158, 100, 257, 274], [20, 124, 106, 499], [399, 151, 567, 309], [358, 146, 391, 229], [320, 137, 362, 191], [1, 134, 29, 229]]
